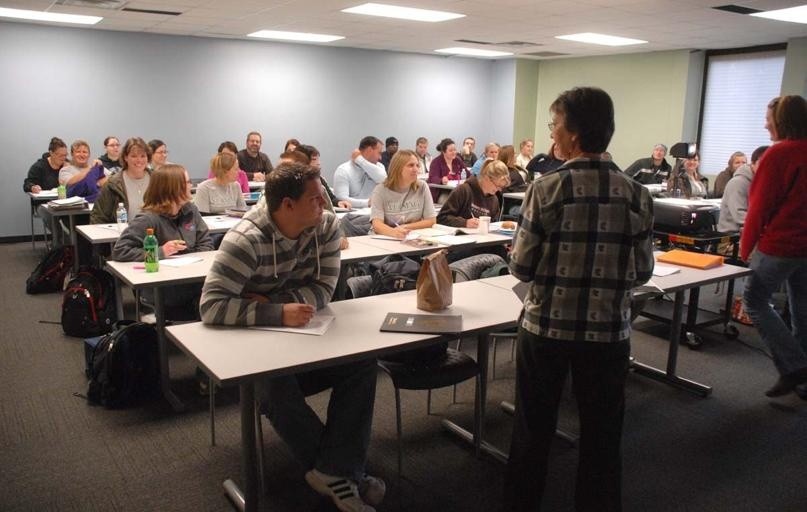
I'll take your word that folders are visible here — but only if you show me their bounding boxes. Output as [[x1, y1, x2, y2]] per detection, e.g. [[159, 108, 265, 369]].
[[656, 248, 726, 270]]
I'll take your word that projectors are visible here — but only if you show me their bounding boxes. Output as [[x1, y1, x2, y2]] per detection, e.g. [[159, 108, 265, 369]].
[[653, 198, 721, 230]]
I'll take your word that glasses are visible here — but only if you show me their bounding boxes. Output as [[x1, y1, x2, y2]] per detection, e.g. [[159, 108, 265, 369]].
[[547, 120, 568, 131]]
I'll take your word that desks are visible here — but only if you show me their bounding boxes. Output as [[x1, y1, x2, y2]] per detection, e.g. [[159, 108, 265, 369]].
[[163, 281, 524, 512], [107, 241, 393, 413], [42, 201, 95, 272], [26, 190, 58, 250], [644, 183, 666, 194], [430, 179, 466, 189], [476, 247, 753, 444], [75, 214, 243, 321], [347, 225, 514, 257], [248, 180, 265, 190], [504, 190, 524, 200]]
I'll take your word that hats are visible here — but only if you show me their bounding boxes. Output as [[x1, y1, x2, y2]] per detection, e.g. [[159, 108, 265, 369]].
[[385, 136, 399, 148]]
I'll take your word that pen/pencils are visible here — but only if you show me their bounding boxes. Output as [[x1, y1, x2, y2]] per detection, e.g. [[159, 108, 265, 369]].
[[300, 294, 308, 304], [392, 220, 402, 229], [172, 239, 184, 246], [471, 212, 475, 218]]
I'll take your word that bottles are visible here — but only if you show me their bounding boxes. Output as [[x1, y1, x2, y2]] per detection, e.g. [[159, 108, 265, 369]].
[[58, 183, 66, 200], [461, 169, 466, 184], [116, 202, 127, 234], [143, 229, 159, 272]]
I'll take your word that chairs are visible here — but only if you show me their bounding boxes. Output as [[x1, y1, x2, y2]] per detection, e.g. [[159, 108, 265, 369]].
[[451, 255, 517, 378], [135, 288, 193, 321], [349, 274, 481, 472], [205, 380, 331, 511]]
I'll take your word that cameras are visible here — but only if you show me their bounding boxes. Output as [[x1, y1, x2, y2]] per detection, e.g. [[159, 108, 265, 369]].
[[669, 142, 698, 158]]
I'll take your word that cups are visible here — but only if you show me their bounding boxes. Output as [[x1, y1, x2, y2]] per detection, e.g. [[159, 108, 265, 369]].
[[477, 216, 491, 234]]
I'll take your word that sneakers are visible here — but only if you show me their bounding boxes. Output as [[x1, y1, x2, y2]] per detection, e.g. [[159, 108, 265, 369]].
[[353, 467, 387, 505], [298, 460, 378, 511], [761, 360, 806, 398]]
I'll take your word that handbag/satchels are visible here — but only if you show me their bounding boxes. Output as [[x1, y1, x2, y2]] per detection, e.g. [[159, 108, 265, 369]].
[[731, 294, 755, 326], [678, 208, 719, 238]]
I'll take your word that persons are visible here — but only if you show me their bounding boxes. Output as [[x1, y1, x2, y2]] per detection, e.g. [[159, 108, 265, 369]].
[[741, 94, 807, 401], [24, 133, 769, 398], [199, 158, 386, 512], [507, 88, 654, 510]]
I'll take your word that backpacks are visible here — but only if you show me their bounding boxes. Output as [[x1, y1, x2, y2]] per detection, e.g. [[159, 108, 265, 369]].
[[85, 316, 159, 410], [25, 243, 82, 295], [369, 252, 450, 360], [61, 267, 118, 337]]
